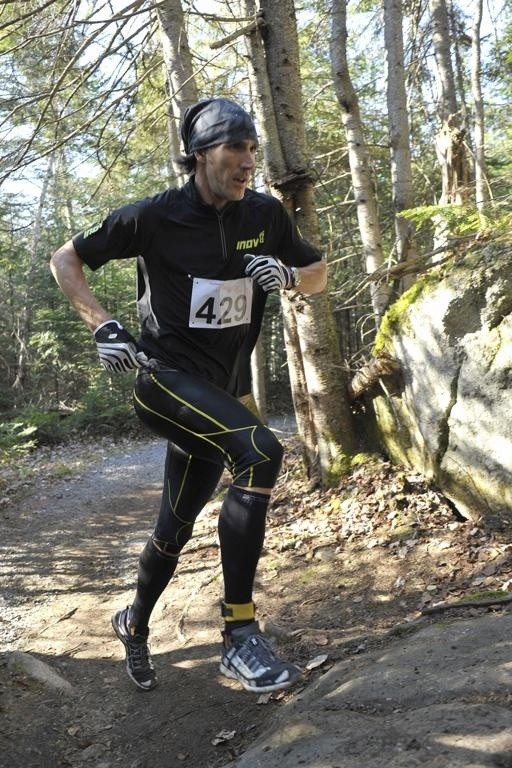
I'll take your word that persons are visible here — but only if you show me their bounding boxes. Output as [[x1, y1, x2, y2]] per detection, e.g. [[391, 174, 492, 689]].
[[49, 96, 329, 695]]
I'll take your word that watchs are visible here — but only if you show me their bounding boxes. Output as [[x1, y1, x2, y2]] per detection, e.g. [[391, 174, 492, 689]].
[[291, 265, 301, 287]]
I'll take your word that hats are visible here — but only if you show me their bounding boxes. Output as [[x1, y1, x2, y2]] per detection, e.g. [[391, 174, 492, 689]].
[[181, 98, 256, 154]]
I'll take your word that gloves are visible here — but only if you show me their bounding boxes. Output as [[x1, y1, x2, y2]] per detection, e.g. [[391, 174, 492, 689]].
[[93, 320, 148, 374], [244, 254, 301, 291]]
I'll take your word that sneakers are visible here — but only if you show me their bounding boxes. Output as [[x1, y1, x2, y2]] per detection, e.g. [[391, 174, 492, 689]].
[[111, 605, 159, 690], [219, 631, 298, 693]]
